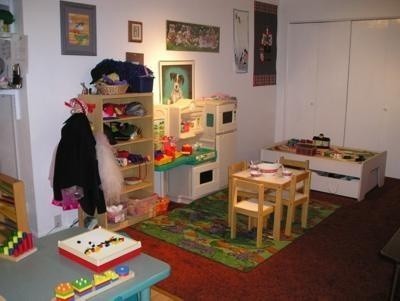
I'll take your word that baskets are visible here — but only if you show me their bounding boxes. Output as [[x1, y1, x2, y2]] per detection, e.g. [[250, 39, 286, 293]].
[[95, 82, 129, 95]]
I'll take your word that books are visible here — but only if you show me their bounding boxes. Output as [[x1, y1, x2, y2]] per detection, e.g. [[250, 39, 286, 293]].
[[57, 228, 141, 265]]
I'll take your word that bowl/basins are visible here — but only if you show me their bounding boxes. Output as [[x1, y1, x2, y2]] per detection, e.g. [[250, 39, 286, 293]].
[[248, 163, 293, 177]]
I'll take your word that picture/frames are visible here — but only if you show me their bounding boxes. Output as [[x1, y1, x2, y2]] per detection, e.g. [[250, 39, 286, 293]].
[[128, 20, 143, 42], [60, 0, 97, 55], [159, 60, 195, 106], [125, 53, 145, 69]]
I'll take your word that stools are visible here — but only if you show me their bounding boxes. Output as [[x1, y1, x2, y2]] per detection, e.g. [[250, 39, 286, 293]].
[[380, 227, 400, 301]]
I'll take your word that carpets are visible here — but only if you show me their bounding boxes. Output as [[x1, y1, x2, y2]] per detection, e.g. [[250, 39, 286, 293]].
[[128, 188, 341, 273]]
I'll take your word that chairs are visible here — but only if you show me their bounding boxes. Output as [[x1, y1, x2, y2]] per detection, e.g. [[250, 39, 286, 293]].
[[230, 178, 275, 246], [265, 169, 312, 238], [279, 156, 310, 193], [227, 160, 271, 224]]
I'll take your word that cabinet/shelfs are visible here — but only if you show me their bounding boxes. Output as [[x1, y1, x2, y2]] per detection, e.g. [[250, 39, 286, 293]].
[[1, 173, 28, 244], [155, 100, 238, 204], [260, 140, 387, 202], [78, 93, 155, 232]]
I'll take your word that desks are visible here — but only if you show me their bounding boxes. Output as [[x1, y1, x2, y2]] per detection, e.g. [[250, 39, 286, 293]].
[[1, 226, 172, 301], [230, 168, 304, 236]]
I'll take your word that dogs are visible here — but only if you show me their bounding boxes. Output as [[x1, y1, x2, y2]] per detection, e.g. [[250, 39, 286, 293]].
[[167, 72, 184, 105]]
